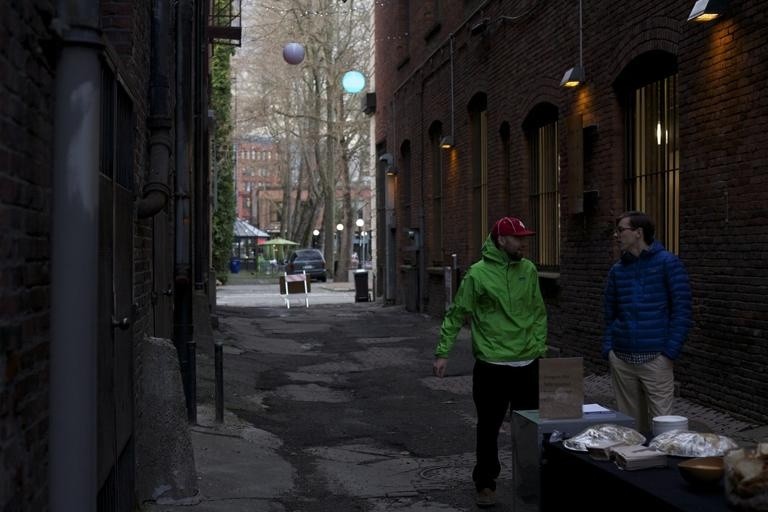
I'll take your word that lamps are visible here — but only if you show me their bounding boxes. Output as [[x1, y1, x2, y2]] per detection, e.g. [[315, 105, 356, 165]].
[[559, 67, 585, 89], [385, 167, 394, 176], [687, 0, 728, 24], [439, 136, 453, 150]]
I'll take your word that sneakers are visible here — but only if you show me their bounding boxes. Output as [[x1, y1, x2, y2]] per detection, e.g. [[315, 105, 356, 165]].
[[477, 487, 497, 506]]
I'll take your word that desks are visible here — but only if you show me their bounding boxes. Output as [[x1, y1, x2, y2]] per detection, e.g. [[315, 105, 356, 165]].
[[540, 438, 767, 512]]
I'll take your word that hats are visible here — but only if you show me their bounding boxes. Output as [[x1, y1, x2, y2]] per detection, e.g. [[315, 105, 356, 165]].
[[492, 217, 536, 236]]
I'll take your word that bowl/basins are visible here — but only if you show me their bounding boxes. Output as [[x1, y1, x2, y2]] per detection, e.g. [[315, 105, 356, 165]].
[[676, 456, 727, 486]]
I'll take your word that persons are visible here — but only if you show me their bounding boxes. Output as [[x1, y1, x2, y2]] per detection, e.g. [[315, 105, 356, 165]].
[[432, 216, 549, 506], [596, 210, 692, 439]]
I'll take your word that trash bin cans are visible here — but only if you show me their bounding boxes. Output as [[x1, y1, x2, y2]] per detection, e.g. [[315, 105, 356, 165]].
[[230, 260, 241, 273], [353, 270, 371, 302]]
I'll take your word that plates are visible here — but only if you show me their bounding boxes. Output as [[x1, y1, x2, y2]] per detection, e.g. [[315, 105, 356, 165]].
[[651, 414, 688, 436]]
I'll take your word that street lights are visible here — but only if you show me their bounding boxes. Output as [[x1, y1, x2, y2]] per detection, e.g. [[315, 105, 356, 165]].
[[360, 231, 367, 266], [354, 218, 365, 269], [312, 229, 320, 250], [335, 223, 345, 255]]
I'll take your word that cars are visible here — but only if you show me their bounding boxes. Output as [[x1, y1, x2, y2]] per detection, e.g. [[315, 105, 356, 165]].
[[284, 248, 327, 284]]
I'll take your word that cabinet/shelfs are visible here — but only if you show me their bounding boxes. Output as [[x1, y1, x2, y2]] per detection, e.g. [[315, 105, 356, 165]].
[[511, 404, 636, 511]]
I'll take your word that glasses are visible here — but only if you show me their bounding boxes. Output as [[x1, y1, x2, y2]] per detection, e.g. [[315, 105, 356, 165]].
[[618, 227, 633, 232]]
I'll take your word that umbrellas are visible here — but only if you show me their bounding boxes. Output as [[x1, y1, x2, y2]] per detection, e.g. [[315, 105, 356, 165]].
[[232, 216, 270, 258], [256, 236, 300, 264]]
